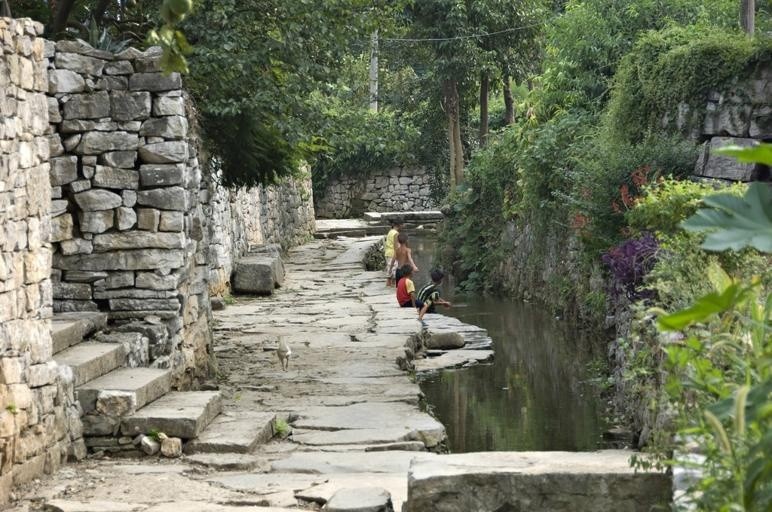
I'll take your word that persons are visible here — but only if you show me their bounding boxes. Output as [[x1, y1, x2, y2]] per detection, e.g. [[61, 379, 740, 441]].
[[387, 234, 421, 288], [382, 216, 406, 288], [396, 264, 432, 326], [416, 269, 451, 314]]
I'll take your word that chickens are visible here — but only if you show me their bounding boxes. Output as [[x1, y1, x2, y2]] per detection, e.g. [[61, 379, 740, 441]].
[[277, 335, 291, 372]]
